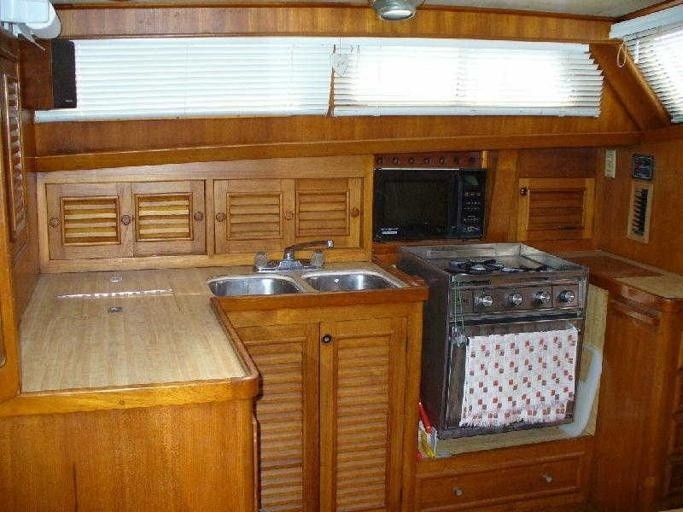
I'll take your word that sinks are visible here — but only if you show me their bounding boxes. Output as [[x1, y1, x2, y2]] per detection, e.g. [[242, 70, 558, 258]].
[[301, 268, 412, 293], [206, 272, 303, 296]]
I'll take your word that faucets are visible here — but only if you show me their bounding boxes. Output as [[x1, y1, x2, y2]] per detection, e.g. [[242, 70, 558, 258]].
[[282, 234, 335, 261]]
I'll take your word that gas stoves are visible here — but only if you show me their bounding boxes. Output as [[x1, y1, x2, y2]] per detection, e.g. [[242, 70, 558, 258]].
[[401, 243, 589, 283]]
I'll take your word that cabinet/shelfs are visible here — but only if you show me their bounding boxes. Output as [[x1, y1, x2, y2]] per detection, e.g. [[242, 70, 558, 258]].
[[1, 153, 683, 511]]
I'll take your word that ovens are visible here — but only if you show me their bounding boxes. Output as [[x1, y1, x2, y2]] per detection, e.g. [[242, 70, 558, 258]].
[[444, 316, 586, 432]]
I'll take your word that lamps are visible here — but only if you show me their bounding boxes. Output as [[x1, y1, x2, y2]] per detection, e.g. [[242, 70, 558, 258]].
[[369, 0, 425, 22]]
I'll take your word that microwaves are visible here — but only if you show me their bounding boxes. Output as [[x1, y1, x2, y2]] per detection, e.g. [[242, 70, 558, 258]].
[[365, 164, 491, 244]]
